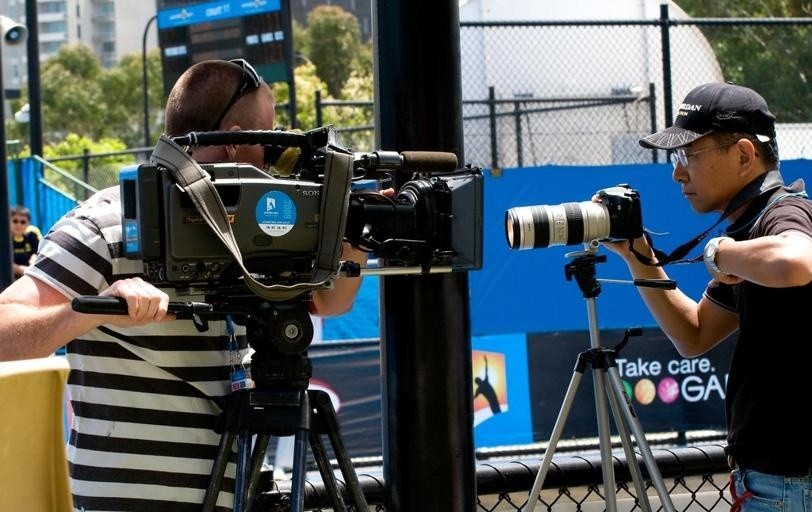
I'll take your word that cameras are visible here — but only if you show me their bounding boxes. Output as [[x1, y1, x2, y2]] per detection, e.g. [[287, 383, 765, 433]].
[[504, 183, 642, 251]]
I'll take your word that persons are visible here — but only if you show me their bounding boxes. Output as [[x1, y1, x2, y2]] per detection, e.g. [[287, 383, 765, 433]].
[[9, 204, 42, 274], [587, 80, 812, 511], [1, 59, 397, 512]]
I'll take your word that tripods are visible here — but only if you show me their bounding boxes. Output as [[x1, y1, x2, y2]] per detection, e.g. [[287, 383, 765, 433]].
[[522, 256, 678, 511], [202, 322, 371, 512]]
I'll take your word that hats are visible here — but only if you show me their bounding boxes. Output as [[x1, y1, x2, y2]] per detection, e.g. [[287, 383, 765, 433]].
[[638, 80, 777, 152]]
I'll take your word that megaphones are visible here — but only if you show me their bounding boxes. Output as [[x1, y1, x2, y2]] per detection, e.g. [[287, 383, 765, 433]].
[[0, 15, 29, 47]]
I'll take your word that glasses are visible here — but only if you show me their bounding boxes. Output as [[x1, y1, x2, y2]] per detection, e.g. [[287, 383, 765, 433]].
[[13, 219, 27, 224], [669, 142, 762, 168], [211, 58, 261, 131]]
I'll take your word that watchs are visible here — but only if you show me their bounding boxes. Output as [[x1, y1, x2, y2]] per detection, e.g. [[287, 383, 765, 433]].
[[702, 233, 736, 277]]
